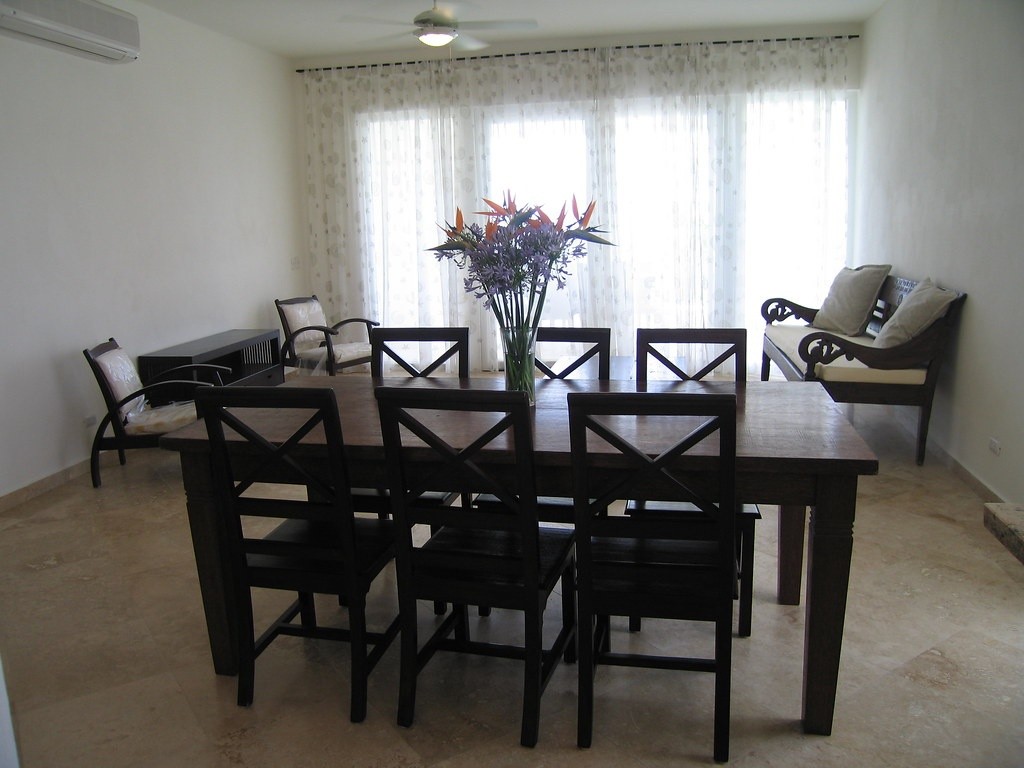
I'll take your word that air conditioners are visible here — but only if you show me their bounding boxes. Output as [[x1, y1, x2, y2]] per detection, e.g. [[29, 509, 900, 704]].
[[0, 0, 141, 64]]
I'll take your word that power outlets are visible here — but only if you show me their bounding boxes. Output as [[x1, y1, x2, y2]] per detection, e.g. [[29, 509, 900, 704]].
[[988, 436, 1000, 456]]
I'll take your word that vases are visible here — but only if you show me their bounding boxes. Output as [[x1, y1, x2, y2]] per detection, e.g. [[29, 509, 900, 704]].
[[499, 327, 538, 406]]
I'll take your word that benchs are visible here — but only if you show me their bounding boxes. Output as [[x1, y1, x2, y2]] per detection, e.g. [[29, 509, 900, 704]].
[[760, 275, 969, 467]]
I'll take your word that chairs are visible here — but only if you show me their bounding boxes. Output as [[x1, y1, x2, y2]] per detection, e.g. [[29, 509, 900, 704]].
[[566, 390, 738, 765], [469, 324, 611, 626], [275, 294, 380, 376], [82, 337, 232, 489], [374, 386, 578, 748], [623, 328, 763, 637], [193, 386, 405, 723], [327, 327, 474, 614]]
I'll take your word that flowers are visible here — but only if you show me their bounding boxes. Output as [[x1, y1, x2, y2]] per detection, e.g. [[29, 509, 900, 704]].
[[423, 190, 617, 404]]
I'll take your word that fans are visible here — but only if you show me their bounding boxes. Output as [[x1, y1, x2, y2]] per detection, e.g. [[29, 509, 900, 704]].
[[338, 0, 540, 54]]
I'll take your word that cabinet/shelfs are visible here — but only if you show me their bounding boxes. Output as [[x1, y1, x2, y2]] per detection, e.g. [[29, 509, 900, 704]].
[[137, 329, 285, 412]]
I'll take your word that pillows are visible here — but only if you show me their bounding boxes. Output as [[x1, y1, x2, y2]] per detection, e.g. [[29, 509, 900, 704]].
[[804, 264, 892, 335], [871, 276, 958, 347]]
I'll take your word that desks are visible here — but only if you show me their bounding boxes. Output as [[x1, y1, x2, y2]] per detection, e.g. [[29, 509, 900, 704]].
[[158, 372, 879, 737]]
